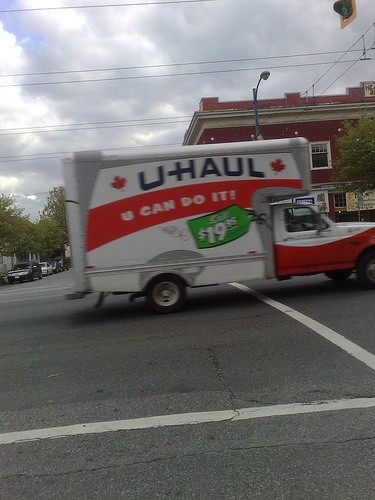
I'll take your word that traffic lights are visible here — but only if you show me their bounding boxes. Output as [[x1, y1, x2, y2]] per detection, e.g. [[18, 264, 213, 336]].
[[333, 0, 357, 30]]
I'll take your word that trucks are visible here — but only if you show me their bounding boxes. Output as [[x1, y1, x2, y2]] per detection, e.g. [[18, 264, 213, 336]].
[[62, 136, 375, 312]]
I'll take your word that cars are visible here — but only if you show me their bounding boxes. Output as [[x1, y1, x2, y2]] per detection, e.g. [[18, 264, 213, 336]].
[[34, 258, 70, 276]]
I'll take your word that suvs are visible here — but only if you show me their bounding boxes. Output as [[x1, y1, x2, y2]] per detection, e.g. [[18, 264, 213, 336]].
[[7, 261, 42, 284]]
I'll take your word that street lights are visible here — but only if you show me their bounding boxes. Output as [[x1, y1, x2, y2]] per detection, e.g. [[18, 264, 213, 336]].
[[252, 71, 270, 140]]
